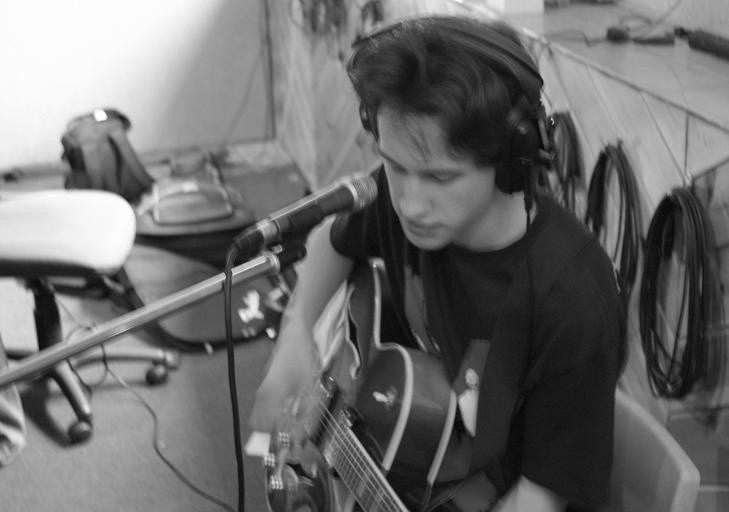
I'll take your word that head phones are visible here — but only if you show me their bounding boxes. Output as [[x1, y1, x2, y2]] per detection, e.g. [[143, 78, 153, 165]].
[[348, 17, 557, 194]]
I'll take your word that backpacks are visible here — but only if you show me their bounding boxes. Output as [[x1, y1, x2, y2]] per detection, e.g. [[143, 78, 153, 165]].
[[61, 108, 155, 205]]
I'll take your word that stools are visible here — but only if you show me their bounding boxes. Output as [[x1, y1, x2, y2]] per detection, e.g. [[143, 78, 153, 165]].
[[0, 168, 174, 444]]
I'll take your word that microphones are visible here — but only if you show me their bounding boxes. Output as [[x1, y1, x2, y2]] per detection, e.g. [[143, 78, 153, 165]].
[[233, 171, 378, 263]]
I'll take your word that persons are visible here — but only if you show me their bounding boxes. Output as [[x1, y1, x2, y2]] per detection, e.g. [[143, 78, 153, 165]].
[[248, 12, 630, 512]]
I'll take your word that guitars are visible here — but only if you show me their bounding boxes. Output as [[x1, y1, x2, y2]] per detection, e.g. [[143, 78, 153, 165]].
[[264, 256, 459, 511]]
